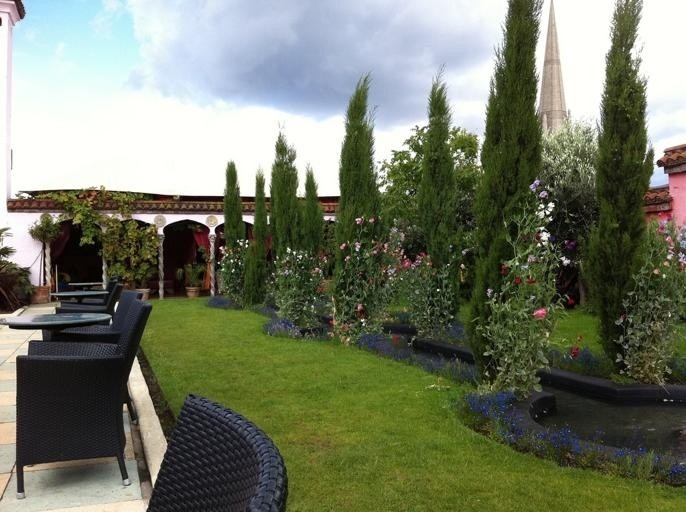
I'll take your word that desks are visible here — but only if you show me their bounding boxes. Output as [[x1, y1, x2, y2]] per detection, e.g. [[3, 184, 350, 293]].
[[9, 313, 112, 341]]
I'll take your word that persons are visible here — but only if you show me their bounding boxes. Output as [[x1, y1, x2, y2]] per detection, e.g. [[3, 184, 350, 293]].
[[58, 271, 72, 288]]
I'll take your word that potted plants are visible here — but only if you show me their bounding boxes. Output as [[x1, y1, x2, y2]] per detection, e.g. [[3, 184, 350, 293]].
[[136, 266, 158, 301], [185, 265, 206, 297], [30, 213, 62, 304]]
